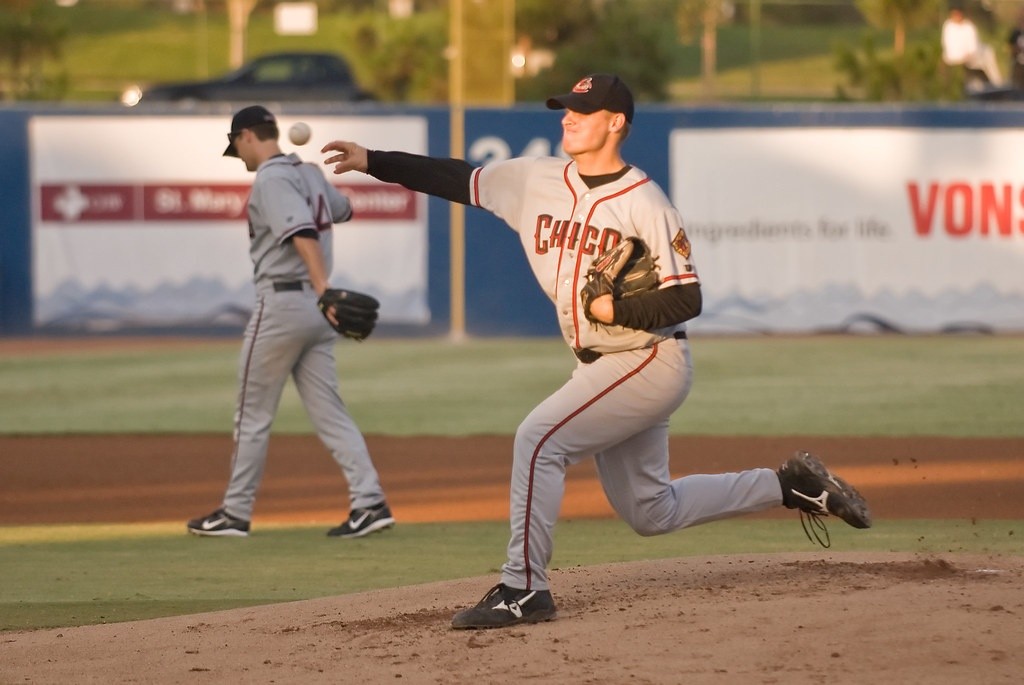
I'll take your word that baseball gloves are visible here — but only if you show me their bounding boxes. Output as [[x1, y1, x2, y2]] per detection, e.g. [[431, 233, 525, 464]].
[[316, 287, 381, 343], [580, 236, 662, 332]]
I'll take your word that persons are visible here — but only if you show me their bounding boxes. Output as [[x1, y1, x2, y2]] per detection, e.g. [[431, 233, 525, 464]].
[[320, 72, 875, 630], [938, 0, 1004, 90], [188, 104, 396, 539]]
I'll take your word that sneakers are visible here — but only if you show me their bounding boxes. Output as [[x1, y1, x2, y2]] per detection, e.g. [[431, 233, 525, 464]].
[[188, 508, 250, 536], [450, 583, 557, 627], [327, 500, 394, 540], [776, 450, 871, 549]]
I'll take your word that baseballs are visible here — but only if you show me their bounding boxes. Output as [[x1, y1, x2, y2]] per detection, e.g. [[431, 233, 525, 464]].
[[288, 122, 310, 145]]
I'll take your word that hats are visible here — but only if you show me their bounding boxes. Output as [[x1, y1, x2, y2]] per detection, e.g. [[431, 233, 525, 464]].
[[546, 72, 634, 124], [222, 104, 276, 157]]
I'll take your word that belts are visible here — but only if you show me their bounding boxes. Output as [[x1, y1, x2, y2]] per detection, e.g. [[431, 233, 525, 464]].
[[273, 280, 304, 291], [575, 331, 688, 365]]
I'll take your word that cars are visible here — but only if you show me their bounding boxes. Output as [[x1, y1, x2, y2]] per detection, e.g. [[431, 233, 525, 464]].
[[121, 51, 379, 112]]
